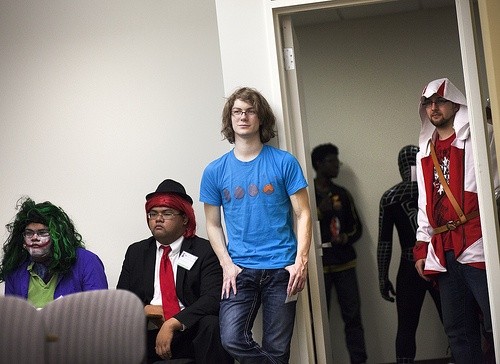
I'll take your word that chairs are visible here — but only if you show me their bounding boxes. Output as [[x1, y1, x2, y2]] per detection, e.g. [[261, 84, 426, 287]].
[[38, 289, 145, 364], [0, 295, 46, 364]]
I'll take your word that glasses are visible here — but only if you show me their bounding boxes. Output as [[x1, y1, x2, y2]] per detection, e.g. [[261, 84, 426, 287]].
[[147, 212, 183, 220], [422, 98, 450, 108], [22, 229, 50, 238], [231, 108, 256, 116]]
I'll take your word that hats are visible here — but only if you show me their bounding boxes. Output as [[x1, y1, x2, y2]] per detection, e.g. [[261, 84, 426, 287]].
[[145, 179, 193, 206]]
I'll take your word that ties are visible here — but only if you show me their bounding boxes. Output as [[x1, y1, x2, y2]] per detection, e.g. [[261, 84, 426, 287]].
[[159, 245, 181, 322]]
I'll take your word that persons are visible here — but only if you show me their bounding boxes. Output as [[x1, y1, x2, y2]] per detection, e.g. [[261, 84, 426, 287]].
[[198, 87, 313, 364], [377, 145, 443, 364], [412, 76, 500, 364], [0, 195, 108, 309], [311, 142, 369, 364], [116, 178, 236, 364]]
